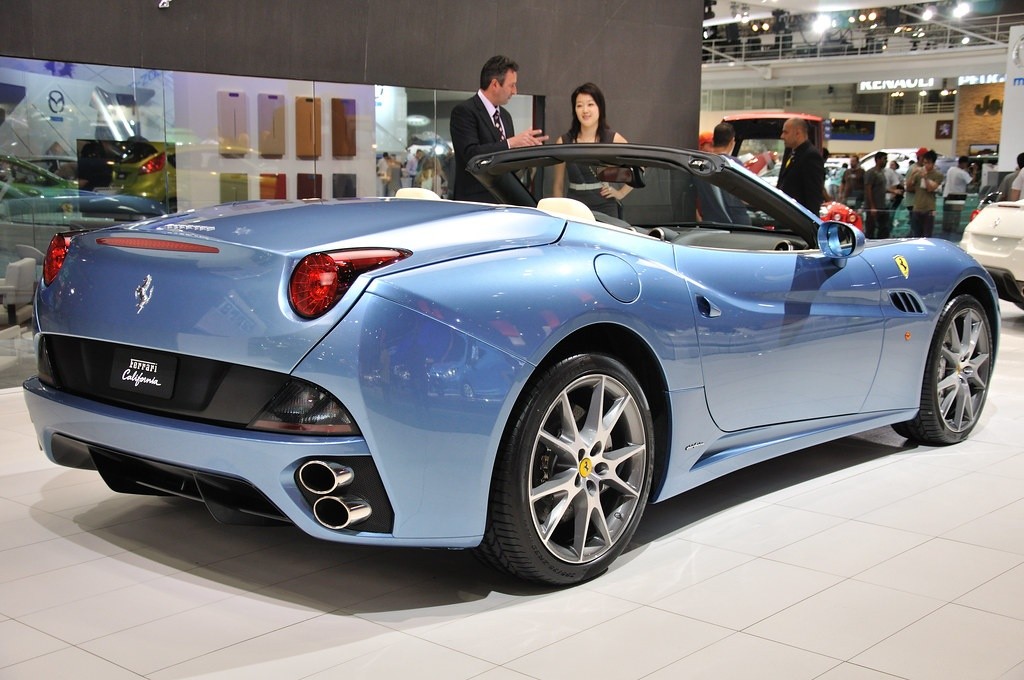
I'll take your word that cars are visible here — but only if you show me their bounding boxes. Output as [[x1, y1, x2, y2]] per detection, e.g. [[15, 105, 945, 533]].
[[110, 135, 279, 216], [969, 190, 1002, 223], [736, 148, 943, 209], [959, 195, 1024, 313], [0, 152, 171, 283]]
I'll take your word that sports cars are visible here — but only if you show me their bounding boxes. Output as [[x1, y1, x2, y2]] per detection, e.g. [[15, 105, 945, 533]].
[[22, 143, 1004, 590]]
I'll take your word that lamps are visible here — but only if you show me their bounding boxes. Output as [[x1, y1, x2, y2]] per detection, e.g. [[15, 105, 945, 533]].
[[752, 20, 762, 32], [730, 2, 741, 21], [762, 19, 773, 32], [703, 26, 715, 39], [741, 3, 751, 23]]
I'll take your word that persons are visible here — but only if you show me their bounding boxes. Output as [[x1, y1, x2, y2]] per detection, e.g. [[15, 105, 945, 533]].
[[552, 83, 646, 219], [377, 148, 457, 200], [696, 118, 1024, 242], [450, 55, 551, 204]]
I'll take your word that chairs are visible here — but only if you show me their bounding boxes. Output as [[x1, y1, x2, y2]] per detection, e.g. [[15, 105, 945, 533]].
[[395, 187, 441, 199], [537, 197, 598, 223]]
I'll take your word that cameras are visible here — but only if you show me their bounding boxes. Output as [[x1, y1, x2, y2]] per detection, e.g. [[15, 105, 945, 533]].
[[968, 167, 973, 171]]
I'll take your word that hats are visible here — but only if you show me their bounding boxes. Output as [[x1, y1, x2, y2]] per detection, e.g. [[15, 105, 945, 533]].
[[916, 148, 928, 156]]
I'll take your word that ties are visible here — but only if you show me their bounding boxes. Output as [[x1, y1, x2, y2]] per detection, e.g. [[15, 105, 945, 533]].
[[493, 111, 506, 142]]
